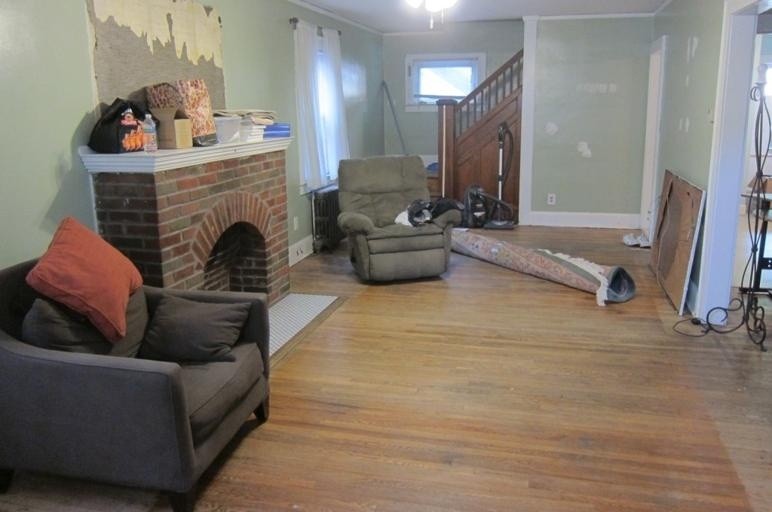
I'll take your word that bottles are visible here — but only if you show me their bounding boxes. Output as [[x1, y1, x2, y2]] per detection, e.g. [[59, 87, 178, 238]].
[[143, 114, 158, 153]]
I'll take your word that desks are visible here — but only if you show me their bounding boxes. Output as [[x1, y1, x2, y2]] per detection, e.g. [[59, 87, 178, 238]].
[[741, 192, 772, 300]]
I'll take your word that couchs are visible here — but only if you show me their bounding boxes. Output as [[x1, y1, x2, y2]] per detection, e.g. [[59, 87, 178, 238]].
[[337, 154, 463, 285], [0, 256, 270, 512]]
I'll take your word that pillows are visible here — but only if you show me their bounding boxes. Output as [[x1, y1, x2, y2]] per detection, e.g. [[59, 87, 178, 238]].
[[25, 215, 143, 341], [135, 290, 252, 363], [22, 288, 151, 358]]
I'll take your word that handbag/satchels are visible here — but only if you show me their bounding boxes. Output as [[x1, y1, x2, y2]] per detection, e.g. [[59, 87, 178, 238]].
[[145, 79, 220, 146], [87, 98, 159, 154]]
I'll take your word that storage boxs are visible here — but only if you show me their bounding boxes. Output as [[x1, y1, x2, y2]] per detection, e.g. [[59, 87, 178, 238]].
[[157, 118, 192, 148], [242, 117, 266, 143], [747, 176, 772, 209], [264, 123, 290, 137], [214, 116, 242, 142]]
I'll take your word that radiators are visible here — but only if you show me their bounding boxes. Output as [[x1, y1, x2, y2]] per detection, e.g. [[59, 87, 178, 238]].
[[311, 185, 339, 254]]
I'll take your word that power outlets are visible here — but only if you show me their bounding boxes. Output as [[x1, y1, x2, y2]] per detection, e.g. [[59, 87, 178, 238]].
[[548, 193, 557, 205]]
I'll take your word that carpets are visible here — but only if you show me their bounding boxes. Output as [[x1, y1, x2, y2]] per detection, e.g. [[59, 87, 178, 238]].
[[269, 290, 349, 369], [452, 227, 637, 306]]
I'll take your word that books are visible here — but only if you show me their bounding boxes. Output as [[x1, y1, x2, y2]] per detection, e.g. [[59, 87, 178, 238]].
[[239, 119, 291, 143]]
